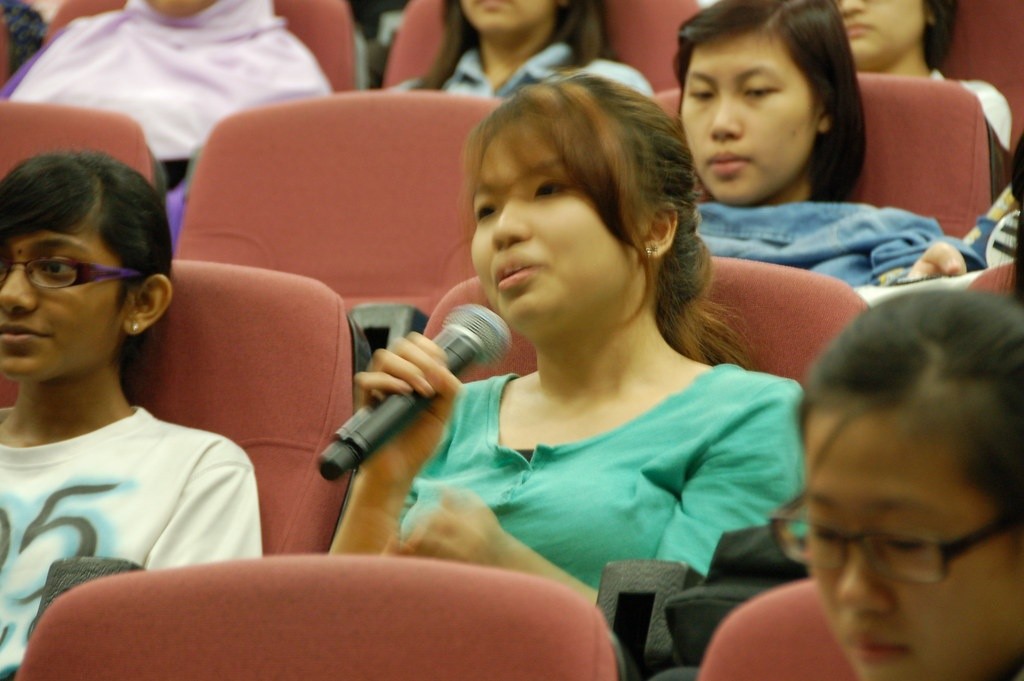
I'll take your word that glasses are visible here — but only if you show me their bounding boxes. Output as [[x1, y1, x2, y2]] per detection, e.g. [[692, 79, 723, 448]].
[[770, 487, 1012, 585], [0, 255, 143, 289]]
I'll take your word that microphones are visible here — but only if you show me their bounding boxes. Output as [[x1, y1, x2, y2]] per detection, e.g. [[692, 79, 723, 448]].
[[316, 305, 510, 481]]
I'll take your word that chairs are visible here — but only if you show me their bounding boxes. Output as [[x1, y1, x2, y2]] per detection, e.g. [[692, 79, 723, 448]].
[[0, 1, 1022, 680]]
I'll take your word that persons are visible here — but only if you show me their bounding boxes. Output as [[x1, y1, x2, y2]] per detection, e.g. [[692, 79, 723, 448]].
[[2, 144, 265, 681], [832, 0, 1013, 156], [670, 0, 947, 291], [1, 0, 337, 264], [895, 131, 1023, 281], [325, 75, 814, 615], [381, 0, 659, 100], [694, 285, 1023, 681]]
